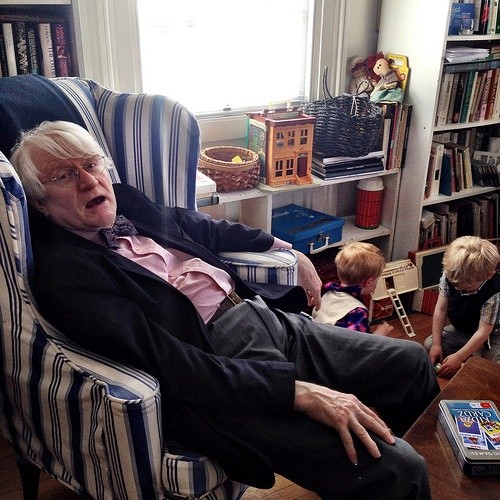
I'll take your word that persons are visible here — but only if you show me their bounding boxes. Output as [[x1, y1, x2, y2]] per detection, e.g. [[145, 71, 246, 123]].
[[8, 121, 440, 500], [312, 242, 394, 338], [423, 236, 500, 378]]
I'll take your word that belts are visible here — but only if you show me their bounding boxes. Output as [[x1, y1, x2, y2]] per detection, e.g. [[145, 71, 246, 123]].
[[209, 292, 243, 325]]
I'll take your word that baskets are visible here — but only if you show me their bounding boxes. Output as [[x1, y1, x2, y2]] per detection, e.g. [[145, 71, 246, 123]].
[[201, 145, 260, 191]]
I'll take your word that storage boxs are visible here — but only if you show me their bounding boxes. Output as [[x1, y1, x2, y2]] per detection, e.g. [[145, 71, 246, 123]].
[[272, 204, 345, 254], [408, 244, 449, 314], [438, 399, 500, 474]]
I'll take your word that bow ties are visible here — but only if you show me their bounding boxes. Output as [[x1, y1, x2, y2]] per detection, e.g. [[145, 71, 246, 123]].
[[98, 214, 138, 248]]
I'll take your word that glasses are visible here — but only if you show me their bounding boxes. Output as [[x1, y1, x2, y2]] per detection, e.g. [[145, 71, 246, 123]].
[[41, 157, 106, 188]]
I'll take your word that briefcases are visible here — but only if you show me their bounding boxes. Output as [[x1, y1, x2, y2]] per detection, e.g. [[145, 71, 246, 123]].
[[272, 204, 345, 254]]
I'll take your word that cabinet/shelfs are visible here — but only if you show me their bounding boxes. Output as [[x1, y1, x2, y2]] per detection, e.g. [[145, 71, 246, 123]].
[[377, 0, 500, 261], [197, 168, 401, 262]]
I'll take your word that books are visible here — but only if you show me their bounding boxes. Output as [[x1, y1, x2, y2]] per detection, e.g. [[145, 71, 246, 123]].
[[310, 103, 413, 181], [0, 13, 73, 78], [195, 170, 220, 206], [419, 0, 500, 249]]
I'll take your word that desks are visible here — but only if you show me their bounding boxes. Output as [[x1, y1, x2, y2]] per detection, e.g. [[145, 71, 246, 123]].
[[402, 355, 500, 500]]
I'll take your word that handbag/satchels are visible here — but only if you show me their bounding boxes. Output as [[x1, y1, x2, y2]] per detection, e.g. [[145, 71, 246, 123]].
[[303, 66, 384, 157]]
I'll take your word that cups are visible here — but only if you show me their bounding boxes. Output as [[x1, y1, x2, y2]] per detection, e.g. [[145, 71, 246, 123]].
[[458, 18, 474, 35]]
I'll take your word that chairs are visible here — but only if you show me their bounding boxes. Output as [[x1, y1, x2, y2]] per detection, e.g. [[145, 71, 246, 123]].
[[0, 77, 299, 500]]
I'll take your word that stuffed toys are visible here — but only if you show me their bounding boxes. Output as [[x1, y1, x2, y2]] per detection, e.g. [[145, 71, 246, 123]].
[[368, 55, 398, 89], [349, 56, 377, 96]]
